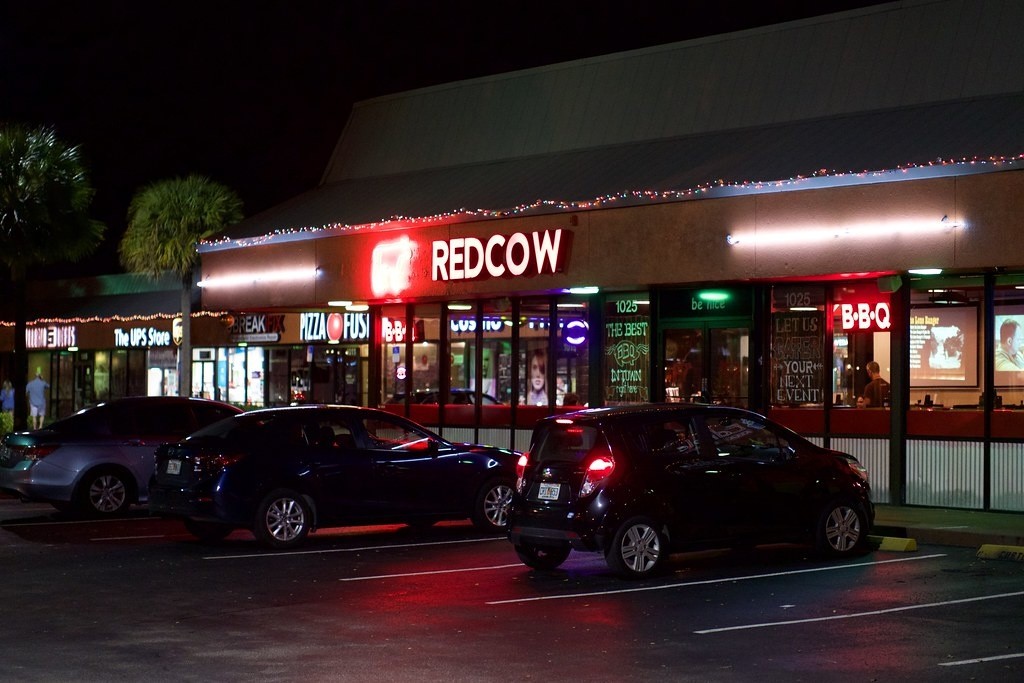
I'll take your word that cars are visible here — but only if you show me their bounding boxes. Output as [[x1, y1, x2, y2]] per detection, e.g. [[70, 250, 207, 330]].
[[504, 402, 876, 582], [384, 388, 501, 405], [0, 396, 267, 521], [146, 403, 529, 554]]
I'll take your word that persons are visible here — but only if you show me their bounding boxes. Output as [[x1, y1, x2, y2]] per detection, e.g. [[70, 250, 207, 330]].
[[0, 380, 14, 412], [995, 319, 1024, 372], [555, 392, 584, 452], [470, 347, 495, 405], [26, 373, 50, 430], [857, 362, 890, 408], [527, 349, 548, 406]]
